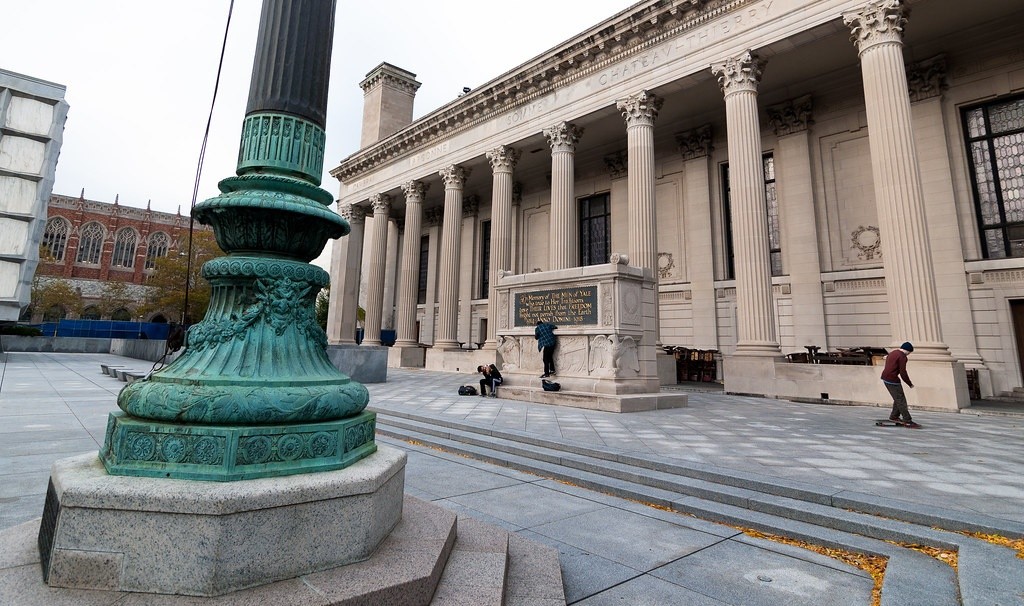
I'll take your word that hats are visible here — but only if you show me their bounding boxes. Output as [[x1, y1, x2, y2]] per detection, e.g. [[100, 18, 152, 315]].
[[900, 342, 913, 352]]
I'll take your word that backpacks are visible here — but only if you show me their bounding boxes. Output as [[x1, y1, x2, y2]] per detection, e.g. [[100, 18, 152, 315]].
[[458, 386, 476, 395], [542, 379, 560, 391]]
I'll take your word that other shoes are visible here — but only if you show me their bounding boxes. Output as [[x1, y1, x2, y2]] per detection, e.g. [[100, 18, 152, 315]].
[[479, 394, 487, 397], [889, 418, 904, 424], [487, 394, 496, 398], [549, 371, 556, 376], [904, 422, 921, 429], [539, 374, 550, 379]]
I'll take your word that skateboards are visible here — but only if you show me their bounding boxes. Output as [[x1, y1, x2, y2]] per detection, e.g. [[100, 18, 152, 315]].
[[871, 418, 922, 430]]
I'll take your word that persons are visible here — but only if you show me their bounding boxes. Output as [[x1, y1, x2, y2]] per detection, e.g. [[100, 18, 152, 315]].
[[534, 320, 558, 378], [880, 341, 915, 424], [477, 364, 503, 398]]
